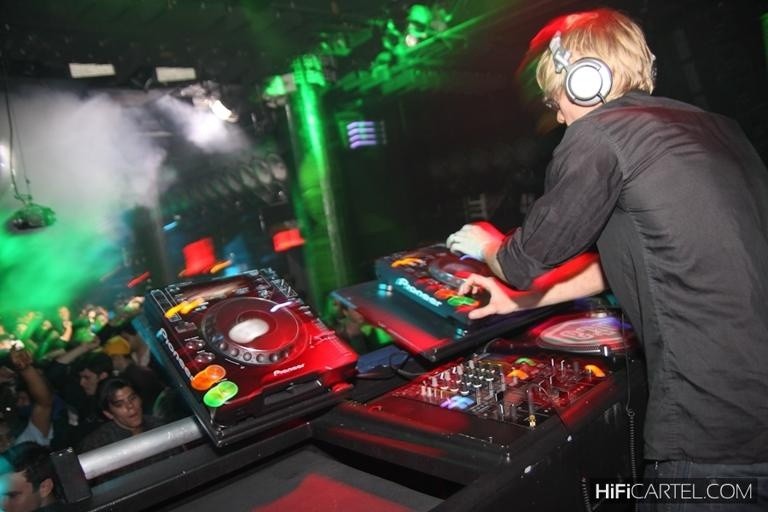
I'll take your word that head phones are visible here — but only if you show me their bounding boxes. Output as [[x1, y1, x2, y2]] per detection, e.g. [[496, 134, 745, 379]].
[[549, 31, 613, 106]]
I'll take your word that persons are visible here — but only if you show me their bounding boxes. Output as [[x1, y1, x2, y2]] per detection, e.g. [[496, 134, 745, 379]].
[[443, 7, 767, 511]]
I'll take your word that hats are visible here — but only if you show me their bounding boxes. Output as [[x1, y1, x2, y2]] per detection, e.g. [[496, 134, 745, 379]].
[[100, 334, 132, 357]]
